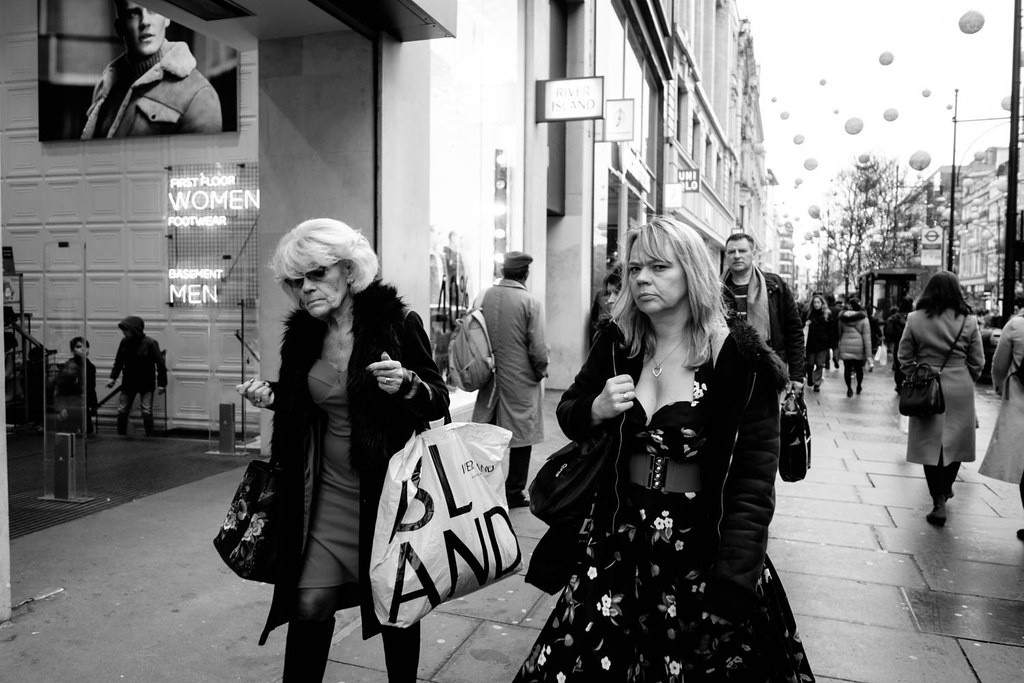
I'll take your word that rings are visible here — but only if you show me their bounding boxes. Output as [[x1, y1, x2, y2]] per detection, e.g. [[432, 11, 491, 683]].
[[384, 376, 391, 383], [254, 398, 261, 403]]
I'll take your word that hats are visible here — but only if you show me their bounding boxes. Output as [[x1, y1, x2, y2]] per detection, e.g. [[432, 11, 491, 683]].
[[504, 251, 533, 268]]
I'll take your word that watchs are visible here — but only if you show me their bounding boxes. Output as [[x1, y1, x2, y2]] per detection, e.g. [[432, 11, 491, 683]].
[[393, 366, 411, 398]]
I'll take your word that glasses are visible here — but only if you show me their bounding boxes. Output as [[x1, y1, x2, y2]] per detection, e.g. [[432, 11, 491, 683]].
[[284, 260, 341, 289]]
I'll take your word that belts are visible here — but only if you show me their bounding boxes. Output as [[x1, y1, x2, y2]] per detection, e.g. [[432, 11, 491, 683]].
[[629, 453, 699, 495]]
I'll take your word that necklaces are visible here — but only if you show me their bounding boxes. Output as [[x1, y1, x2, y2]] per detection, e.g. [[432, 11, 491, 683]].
[[644, 329, 691, 378]]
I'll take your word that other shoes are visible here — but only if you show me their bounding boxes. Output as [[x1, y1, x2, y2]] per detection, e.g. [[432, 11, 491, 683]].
[[814, 385, 820, 391], [825, 359, 830, 369], [927, 495, 947, 526], [808, 374, 814, 386], [833, 357, 839, 368]]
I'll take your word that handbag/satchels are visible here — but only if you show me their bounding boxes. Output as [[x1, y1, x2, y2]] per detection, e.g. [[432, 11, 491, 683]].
[[529, 440, 613, 526], [899, 363, 945, 415], [213, 460, 282, 585], [803, 326, 809, 346], [779, 391, 811, 482], [874, 340, 888, 366], [368, 384, 525, 629]]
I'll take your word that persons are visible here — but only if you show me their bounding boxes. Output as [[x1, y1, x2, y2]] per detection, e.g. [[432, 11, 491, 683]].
[[978, 286, 1024, 541], [105, 315, 168, 436], [17, 337, 99, 436], [233, 218, 451, 683], [470, 250, 549, 508], [896, 272, 985, 524], [533, 218, 815, 682], [796, 291, 915, 397], [78, 2, 224, 141]]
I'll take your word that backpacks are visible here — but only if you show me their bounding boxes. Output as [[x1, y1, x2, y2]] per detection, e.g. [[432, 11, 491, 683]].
[[448, 289, 495, 392]]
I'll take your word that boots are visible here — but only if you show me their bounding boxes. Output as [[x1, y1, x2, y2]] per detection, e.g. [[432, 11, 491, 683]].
[[117, 414, 129, 437], [143, 415, 155, 437]]
